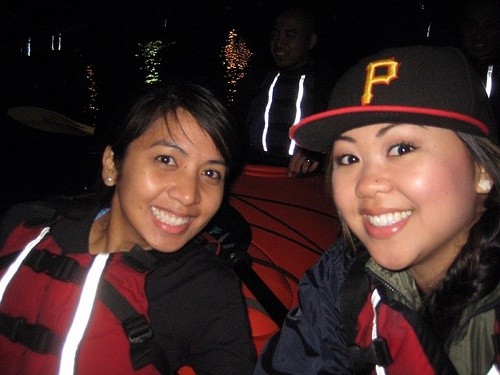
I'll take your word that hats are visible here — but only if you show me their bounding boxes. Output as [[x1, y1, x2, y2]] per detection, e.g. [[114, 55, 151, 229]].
[[288, 43, 494, 154]]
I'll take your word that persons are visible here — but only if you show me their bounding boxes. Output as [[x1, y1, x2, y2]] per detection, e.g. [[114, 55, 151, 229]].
[[232, 5, 349, 177], [1, 84, 255, 375], [254, 43, 500, 375]]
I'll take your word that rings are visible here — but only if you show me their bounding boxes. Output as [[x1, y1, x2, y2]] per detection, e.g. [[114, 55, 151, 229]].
[[304, 156, 312, 166]]
